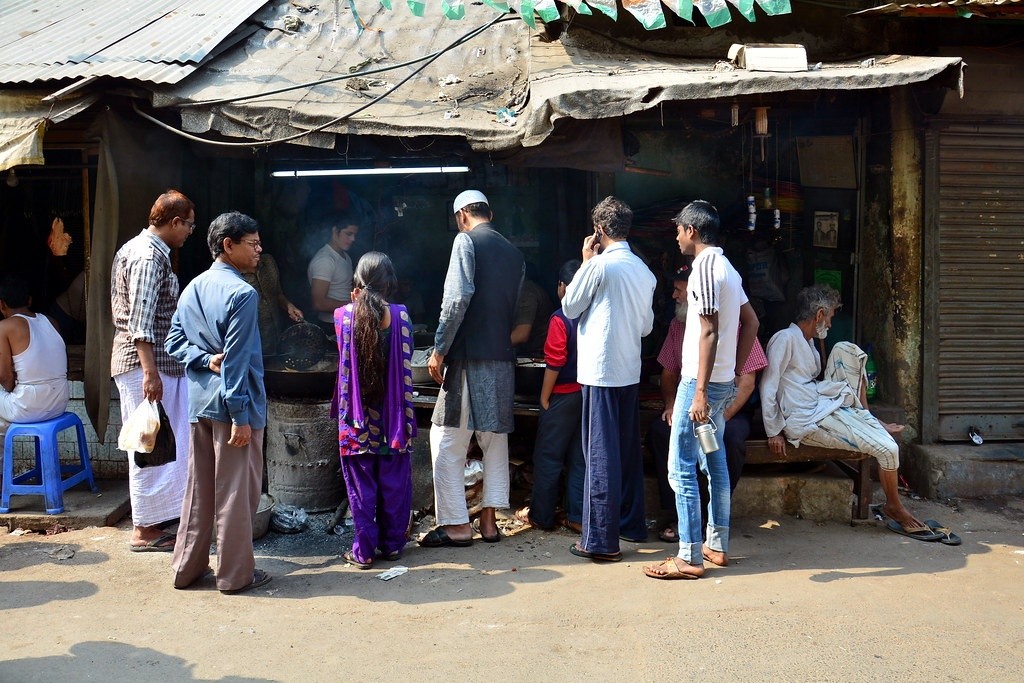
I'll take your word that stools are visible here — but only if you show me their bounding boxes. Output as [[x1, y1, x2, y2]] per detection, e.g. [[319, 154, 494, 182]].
[[0, 412, 99, 516]]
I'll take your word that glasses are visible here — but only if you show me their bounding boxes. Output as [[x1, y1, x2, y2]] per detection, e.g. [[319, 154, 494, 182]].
[[172, 216, 196, 231], [236, 240, 261, 250]]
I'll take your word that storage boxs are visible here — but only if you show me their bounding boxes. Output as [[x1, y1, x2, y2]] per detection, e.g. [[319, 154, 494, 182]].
[[727, 43, 808, 72]]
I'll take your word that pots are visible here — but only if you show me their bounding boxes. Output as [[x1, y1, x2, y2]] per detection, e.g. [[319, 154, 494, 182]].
[[514, 357, 546, 400], [262, 353, 339, 400]]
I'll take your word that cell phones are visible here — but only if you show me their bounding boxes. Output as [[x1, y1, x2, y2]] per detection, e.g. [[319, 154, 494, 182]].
[[591, 229, 602, 250]]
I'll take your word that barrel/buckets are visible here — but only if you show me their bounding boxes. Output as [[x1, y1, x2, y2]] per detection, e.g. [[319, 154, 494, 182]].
[[265, 401, 344, 511]]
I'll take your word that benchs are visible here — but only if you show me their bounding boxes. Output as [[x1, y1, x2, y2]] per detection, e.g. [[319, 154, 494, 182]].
[[640, 438, 875, 520]]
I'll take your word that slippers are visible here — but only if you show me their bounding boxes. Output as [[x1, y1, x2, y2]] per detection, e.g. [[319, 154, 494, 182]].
[[478, 524, 501, 542], [130, 532, 176, 551], [887, 520, 944, 540], [569, 543, 623, 561], [641, 556, 700, 578], [659, 525, 681, 542], [220, 568, 273, 596], [701, 546, 728, 566], [925, 519, 961, 544], [416, 526, 474, 547], [618, 533, 643, 541], [173, 566, 214, 589]]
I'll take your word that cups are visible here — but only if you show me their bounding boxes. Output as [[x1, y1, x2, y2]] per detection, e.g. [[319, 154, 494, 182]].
[[693, 415, 720, 454]]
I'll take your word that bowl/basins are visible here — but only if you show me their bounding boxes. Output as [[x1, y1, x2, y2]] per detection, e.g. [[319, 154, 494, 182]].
[[211, 492, 275, 542], [410, 363, 444, 383]]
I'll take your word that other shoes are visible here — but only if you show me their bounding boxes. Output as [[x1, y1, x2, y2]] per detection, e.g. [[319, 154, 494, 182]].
[[557, 516, 583, 534], [516, 507, 536, 525]]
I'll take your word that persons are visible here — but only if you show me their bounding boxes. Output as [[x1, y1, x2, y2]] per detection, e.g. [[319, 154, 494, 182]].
[[657, 259, 769, 543], [643, 200, 760, 579], [107, 187, 211, 553], [229, 212, 358, 365], [0, 277, 69, 497], [333, 250, 415, 571], [758, 283, 927, 534], [163, 212, 270, 596], [416, 188, 524, 550], [560, 195, 658, 562], [515, 258, 581, 536]]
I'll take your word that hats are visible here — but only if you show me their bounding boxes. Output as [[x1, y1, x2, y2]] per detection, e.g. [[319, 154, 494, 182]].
[[672, 265, 692, 282], [453, 190, 490, 214]]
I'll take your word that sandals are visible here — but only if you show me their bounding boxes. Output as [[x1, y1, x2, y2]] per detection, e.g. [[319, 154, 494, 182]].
[[381, 550, 401, 560], [342, 550, 372, 568]]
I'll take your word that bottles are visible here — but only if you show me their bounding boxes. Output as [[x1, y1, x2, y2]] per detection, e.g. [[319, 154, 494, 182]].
[[865, 352, 878, 404]]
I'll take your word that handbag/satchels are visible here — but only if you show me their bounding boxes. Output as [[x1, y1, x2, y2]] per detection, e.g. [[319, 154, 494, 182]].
[[116, 397, 177, 469]]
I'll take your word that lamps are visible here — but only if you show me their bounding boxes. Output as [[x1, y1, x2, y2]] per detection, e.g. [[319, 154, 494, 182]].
[[271, 158, 471, 177]]
